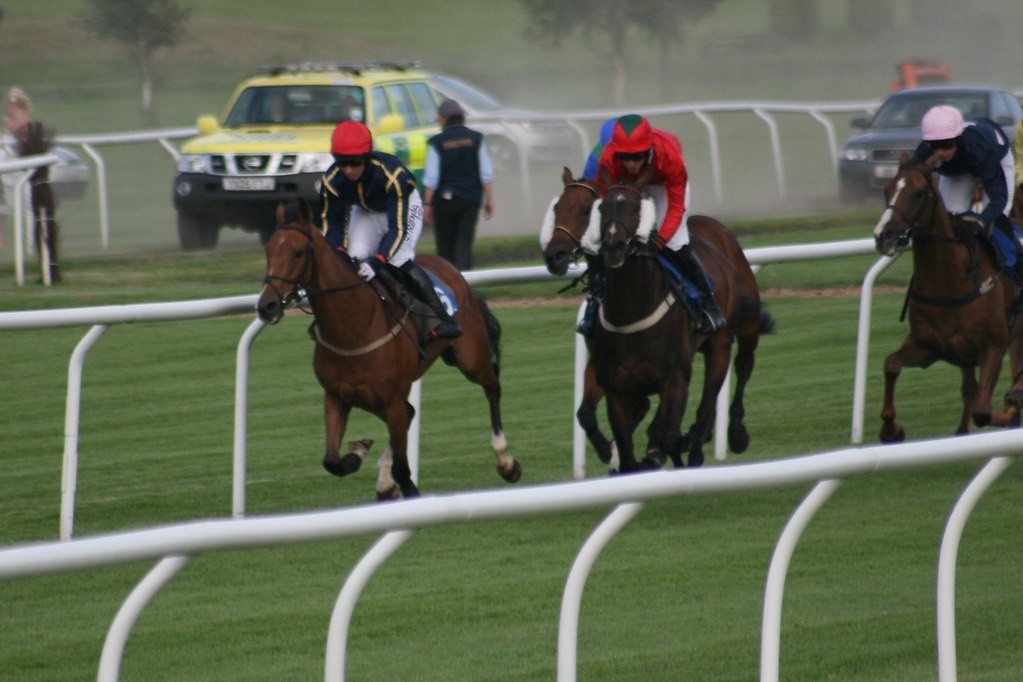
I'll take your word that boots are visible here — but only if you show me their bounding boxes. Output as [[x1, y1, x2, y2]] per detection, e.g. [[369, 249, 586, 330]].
[[663, 244, 727, 328], [399, 259, 462, 346]]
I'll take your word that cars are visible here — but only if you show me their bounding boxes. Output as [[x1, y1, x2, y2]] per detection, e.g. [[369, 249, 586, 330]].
[[425, 73, 576, 177], [840, 85, 1023, 207]]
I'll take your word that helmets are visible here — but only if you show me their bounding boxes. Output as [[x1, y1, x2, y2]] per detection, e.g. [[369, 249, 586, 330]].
[[329, 121, 373, 155], [920, 105, 964, 141]]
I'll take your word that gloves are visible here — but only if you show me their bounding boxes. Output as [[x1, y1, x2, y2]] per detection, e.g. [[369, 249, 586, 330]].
[[648, 236, 667, 253], [357, 256, 384, 286], [962, 219, 986, 237]]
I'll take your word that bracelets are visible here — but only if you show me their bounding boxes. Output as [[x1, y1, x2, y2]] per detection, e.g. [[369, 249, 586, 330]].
[[424, 201, 430, 208]]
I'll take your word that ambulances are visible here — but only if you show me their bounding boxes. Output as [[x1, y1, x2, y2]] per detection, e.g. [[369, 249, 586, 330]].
[[172, 72, 452, 253]]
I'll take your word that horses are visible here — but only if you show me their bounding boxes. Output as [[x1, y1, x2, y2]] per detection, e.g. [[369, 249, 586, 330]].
[[872, 146, 1023, 444], [541, 162, 776, 478], [257, 194, 521, 504]]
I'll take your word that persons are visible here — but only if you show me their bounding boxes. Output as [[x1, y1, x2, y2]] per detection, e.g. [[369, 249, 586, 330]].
[[577, 114, 727, 336], [422, 99, 494, 271], [316, 121, 464, 341], [911, 105, 1023, 313], [3, 86, 64, 285], [581, 117, 618, 181], [1014, 101, 1023, 189]]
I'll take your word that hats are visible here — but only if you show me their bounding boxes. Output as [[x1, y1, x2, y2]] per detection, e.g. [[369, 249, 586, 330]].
[[611, 114, 653, 154], [437, 99, 465, 117]]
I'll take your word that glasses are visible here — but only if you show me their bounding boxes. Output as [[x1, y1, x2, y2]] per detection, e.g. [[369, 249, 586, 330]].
[[926, 140, 954, 151], [615, 151, 646, 163], [334, 157, 364, 168]]
